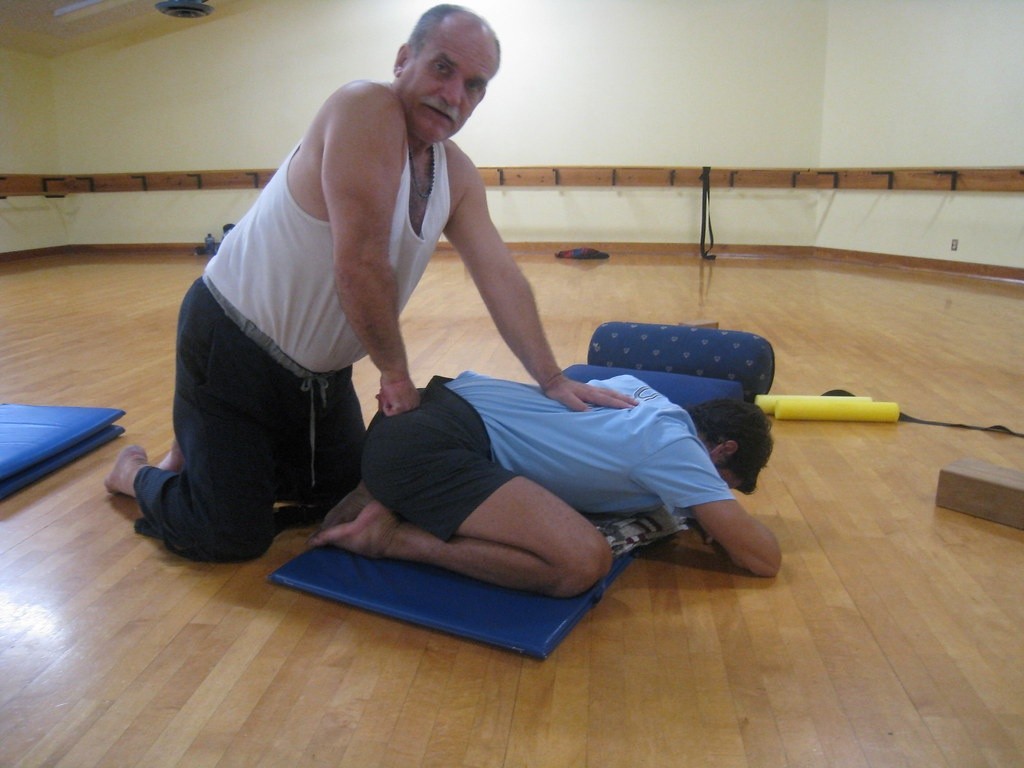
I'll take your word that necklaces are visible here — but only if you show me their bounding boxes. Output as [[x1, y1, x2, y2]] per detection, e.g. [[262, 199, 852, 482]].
[[408, 144, 436, 198]]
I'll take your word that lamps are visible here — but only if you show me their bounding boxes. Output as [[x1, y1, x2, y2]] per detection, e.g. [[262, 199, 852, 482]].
[[155, 0, 215, 18]]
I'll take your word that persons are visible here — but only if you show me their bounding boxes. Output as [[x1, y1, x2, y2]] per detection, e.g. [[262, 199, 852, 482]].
[[103, 5, 639, 567], [307, 370, 781, 596]]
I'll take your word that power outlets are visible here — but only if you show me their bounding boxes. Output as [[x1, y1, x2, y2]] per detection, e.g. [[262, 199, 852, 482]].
[[951, 239, 958, 251]]
